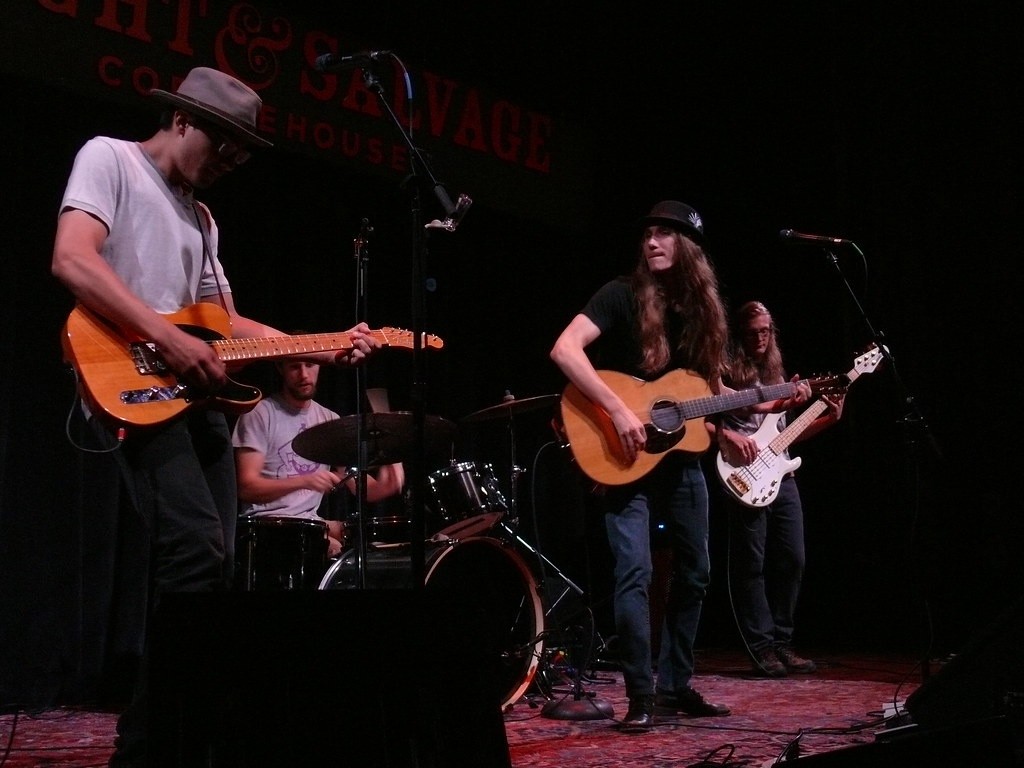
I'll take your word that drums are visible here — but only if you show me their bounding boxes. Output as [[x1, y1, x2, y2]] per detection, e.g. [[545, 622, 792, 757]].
[[238, 511, 332, 589], [345, 509, 418, 540], [319, 534, 548, 712], [408, 457, 509, 542]]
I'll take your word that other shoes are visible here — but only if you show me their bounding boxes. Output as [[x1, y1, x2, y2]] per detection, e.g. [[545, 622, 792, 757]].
[[779, 649, 815, 674], [755, 650, 787, 679], [620, 696, 654, 732], [653, 685, 731, 716]]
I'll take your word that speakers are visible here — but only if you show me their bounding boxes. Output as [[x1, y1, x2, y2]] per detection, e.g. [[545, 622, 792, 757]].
[[772, 714, 1024, 768], [583, 492, 674, 676], [905, 596, 1024, 747], [141, 586, 511, 768]]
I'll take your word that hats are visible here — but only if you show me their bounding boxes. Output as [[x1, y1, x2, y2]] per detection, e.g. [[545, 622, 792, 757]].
[[635, 199, 709, 242], [150, 67, 275, 147]]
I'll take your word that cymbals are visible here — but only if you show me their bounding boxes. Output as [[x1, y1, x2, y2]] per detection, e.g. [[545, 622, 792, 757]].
[[462, 392, 564, 432], [289, 412, 453, 469]]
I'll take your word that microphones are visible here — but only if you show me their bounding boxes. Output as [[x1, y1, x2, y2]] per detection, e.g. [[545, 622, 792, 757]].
[[779, 228, 853, 247], [315, 49, 392, 74], [500, 650, 527, 662]]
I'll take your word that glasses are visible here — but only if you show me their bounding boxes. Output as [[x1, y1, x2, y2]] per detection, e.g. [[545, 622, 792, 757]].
[[742, 327, 770, 340], [189, 111, 249, 166]]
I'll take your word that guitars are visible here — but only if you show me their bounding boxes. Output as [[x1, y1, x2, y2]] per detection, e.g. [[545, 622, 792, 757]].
[[561, 365, 853, 493], [716, 343, 891, 508], [59, 298, 444, 431]]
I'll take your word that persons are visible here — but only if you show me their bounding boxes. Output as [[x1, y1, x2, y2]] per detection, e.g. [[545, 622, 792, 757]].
[[551, 200, 812, 729], [51, 66, 383, 593], [704, 301, 846, 676], [234, 329, 406, 557]]
